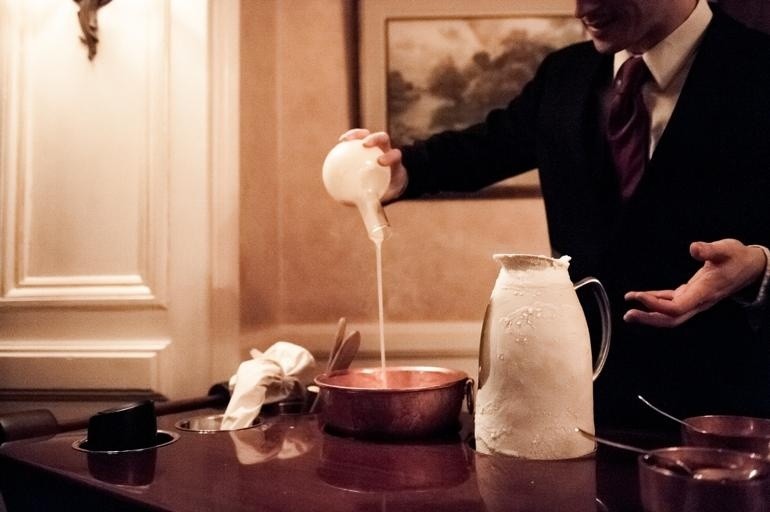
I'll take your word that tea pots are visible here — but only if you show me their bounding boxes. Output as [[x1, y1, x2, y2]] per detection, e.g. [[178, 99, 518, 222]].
[[475, 251, 612, 462]]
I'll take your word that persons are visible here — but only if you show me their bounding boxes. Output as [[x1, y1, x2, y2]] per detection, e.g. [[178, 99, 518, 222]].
[[339, 0, 770, 445]]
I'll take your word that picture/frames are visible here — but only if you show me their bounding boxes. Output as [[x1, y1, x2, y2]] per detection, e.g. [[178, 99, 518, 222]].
[[382, 15, 590, 201]]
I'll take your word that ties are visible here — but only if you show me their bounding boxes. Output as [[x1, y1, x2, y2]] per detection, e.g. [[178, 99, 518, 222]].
[[603, 55, 652, 201]]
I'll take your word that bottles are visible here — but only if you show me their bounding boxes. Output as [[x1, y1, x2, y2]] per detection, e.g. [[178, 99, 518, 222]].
[[322, 138, 391, 243]]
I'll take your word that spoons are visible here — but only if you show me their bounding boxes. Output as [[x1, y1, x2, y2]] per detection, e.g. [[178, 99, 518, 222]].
[[573, 426, 761, 483]]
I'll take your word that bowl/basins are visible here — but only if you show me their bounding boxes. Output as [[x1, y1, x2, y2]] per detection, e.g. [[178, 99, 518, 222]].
[[638, 447, 770, 512], [678, 415, 770, 461], [313, 364, 476, 440]]
